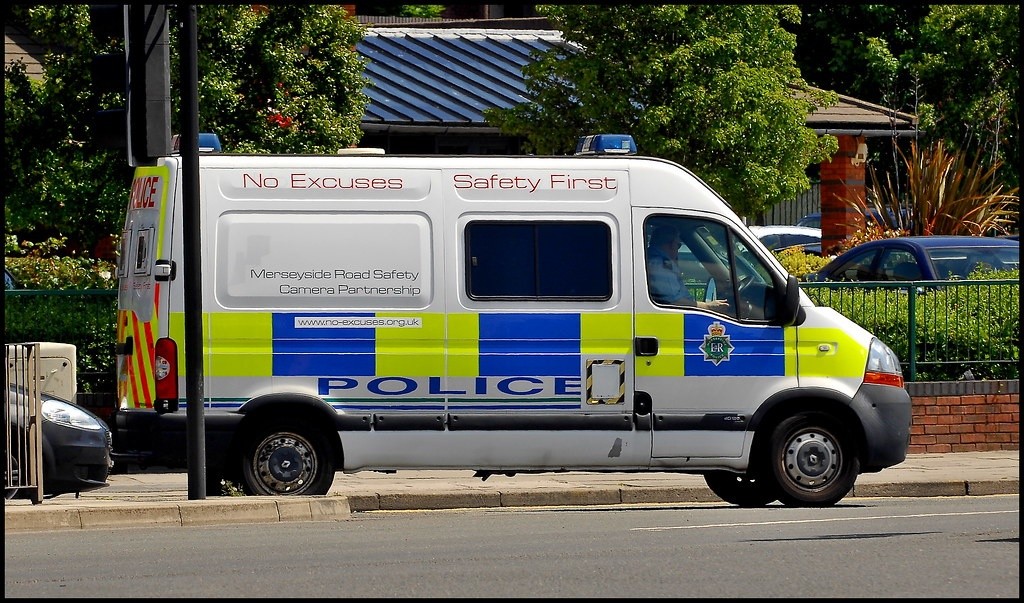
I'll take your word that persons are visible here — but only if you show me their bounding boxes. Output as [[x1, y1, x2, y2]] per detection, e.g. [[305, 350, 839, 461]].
[[646, 222, 731, 312]]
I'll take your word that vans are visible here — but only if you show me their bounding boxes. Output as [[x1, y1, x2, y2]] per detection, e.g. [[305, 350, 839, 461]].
[[110, 132, 912, 508]]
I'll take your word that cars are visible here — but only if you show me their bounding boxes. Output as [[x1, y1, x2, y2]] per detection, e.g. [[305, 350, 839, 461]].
[[741, 223, 822, 258], [802, 235, 1020, 279], [800, 208, 917, 234], [5, 381, 113, 497]]
